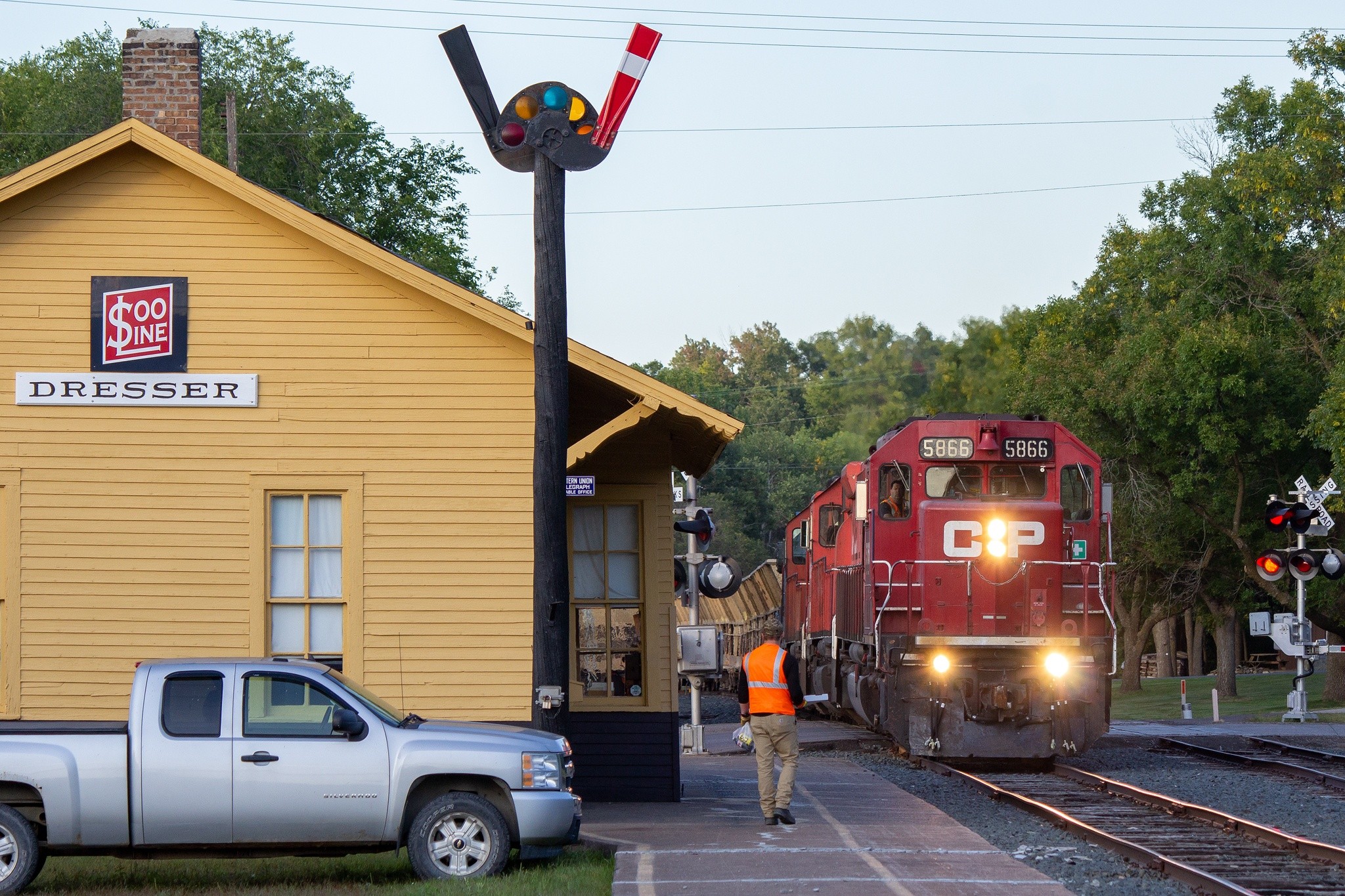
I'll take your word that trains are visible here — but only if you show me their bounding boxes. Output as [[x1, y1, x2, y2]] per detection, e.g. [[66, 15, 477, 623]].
[[674, 412, 1118, 763]]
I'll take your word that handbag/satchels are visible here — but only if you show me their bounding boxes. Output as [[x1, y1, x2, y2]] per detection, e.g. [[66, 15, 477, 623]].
[[732, 721, 756, 755]]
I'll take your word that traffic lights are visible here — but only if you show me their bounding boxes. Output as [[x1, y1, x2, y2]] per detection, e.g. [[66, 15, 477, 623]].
[[673, 558, 687, 599], [1256, 550, 1286, 581], [1264, 501, 1295, 533], [1291, 503, 1320, 534], [1286, 548, 1320, 581], [674, 510, 712, 553]]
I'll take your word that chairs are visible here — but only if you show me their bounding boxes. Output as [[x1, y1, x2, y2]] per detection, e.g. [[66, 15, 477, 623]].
[[202, 691, 221, 735]]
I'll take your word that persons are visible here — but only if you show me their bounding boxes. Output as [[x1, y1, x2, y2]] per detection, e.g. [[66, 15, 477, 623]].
[[879, 480, 909, 518], [738, 619, 807, 825]]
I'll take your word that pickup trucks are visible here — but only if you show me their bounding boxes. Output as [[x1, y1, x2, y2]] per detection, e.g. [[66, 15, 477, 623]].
[[0, 655, 583, 896]]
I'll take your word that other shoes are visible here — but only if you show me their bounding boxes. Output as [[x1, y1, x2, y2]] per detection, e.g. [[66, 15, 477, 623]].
[[773, 808, 796, 825], [764, 817, 779, 825]]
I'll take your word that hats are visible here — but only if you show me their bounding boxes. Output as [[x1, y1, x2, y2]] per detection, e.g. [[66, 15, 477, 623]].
[[763, 618, 784, 636]]
